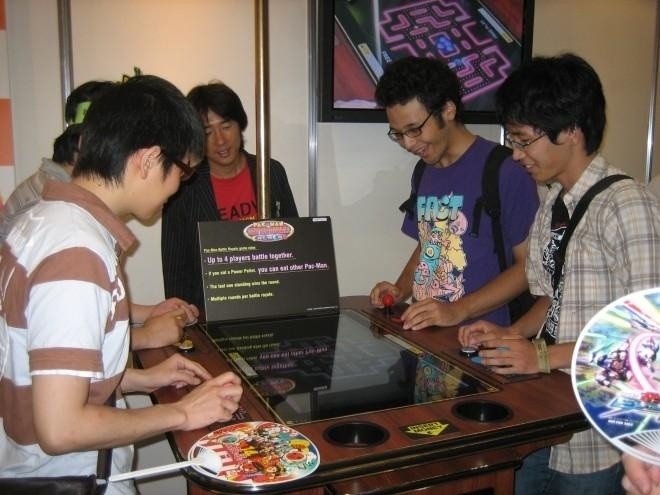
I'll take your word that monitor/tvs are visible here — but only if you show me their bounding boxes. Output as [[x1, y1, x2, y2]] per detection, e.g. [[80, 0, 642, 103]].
[[198, 305, 503, 430]]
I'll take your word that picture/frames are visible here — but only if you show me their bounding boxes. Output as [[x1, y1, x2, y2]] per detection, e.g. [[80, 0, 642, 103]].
[[312, 0, 538, 124]]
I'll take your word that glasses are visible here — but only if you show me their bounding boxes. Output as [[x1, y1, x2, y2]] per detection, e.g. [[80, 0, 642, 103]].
[[386, 112, 434, 142], [503, 131, 547, 151], [163, 151, 197, 184]]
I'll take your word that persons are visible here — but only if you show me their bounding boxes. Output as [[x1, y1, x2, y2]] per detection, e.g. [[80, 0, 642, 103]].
[[370, 56, 541, 332], [458, 52, 660, 495], [0, 81, 121, 249], [621, 446, 660, 495], [0, 76, 244, 494], [160, 79, 299, 325]]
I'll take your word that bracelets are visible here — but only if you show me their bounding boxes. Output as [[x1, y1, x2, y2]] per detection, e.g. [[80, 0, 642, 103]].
[[531, 338, 551, 375]]
